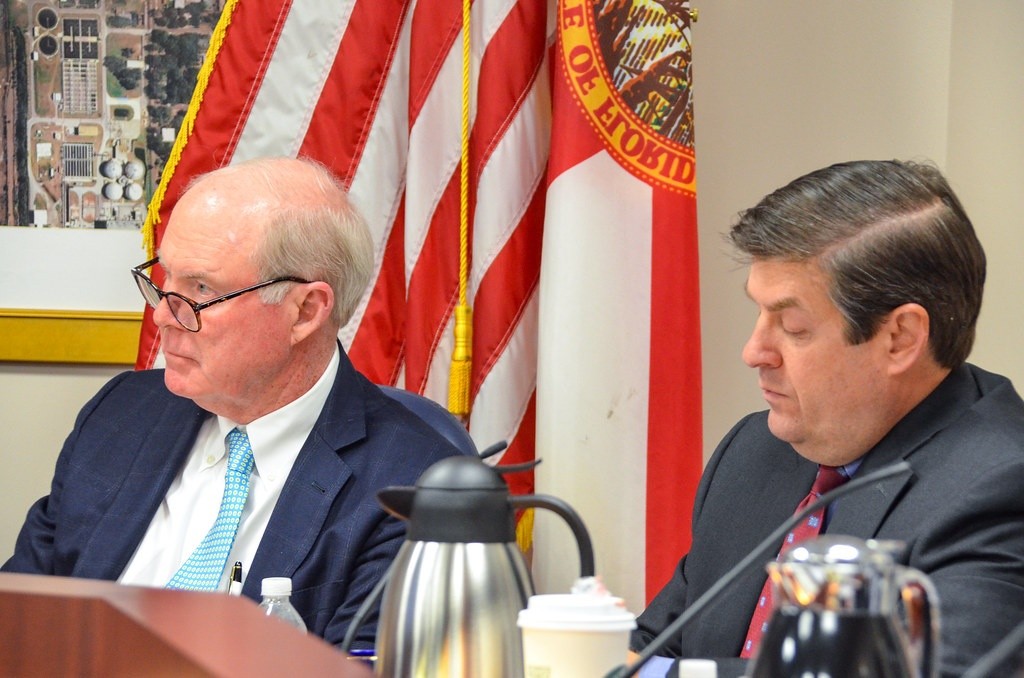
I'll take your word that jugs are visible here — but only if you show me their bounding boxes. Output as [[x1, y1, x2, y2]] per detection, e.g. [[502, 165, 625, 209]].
[[377, 457, 597, 678], [743, 536, 943, 678]]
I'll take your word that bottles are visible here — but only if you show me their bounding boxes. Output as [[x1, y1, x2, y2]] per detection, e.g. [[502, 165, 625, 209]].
[[259, 576, 307, 632]]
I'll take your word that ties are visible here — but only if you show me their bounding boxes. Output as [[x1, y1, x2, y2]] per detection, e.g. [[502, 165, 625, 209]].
[[739, 462, 846, 661], [164, 429, 254, 593]]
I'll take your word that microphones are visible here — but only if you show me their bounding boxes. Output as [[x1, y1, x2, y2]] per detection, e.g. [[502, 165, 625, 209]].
[[620, 463, 912, 678], [342, 440, 508, 653]]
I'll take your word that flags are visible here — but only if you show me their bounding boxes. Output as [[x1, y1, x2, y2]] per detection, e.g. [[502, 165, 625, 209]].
[[133, 0, 703, 618]]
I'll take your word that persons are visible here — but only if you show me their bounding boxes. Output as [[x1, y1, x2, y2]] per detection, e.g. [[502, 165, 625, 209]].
[[627, 160, 1024, 678], [0, 156, 465, 670]]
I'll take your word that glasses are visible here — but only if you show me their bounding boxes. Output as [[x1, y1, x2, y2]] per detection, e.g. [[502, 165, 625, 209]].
[[130, 256, 315, 332]]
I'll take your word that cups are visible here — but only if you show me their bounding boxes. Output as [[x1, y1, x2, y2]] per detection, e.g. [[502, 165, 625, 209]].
[[515, 591, 638, 678]]
